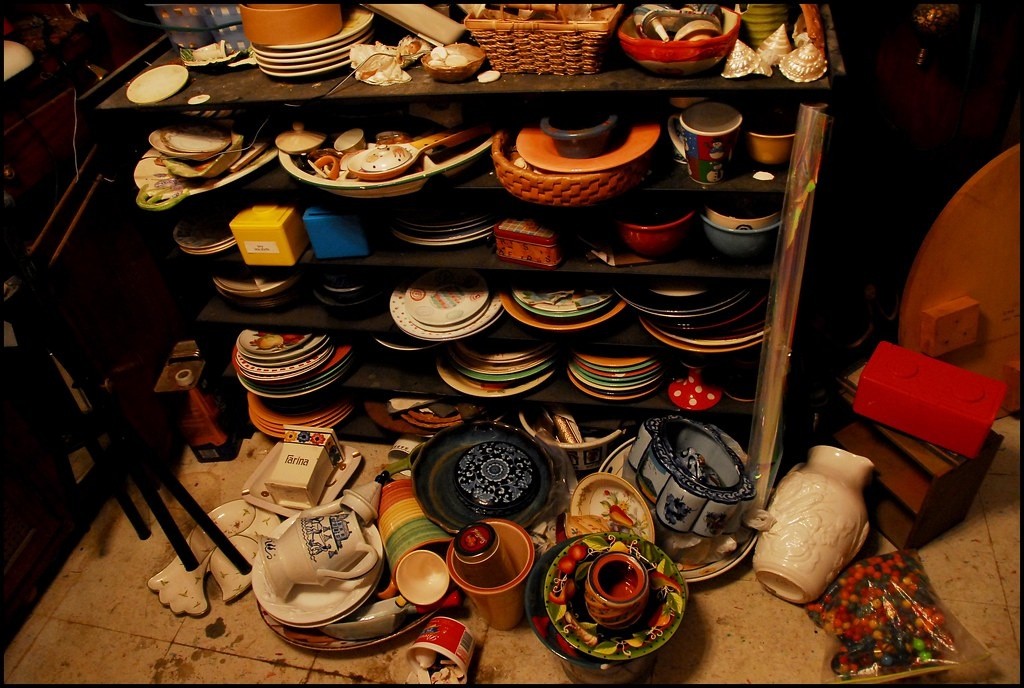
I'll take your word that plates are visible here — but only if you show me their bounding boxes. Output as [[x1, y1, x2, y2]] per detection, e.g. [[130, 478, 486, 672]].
[[499, 287, 627, 333], [599, 436, 760, 583], [391, 214, 499, 246], [411, 420, 557, 534], [389, 269, 505, 342], [251, 512, 440, 651], [613, 289, 767, 353], [126, 64, 189, 104], [134, 141, 278, 211], [569, 473, 656, 545], [242, 438, 361, 518], [437, 345, 561, 398], [250, 8, 375, 77], [246, 392, 354, 438], [160, 127, 231, 153], [566, 352, 668, 401], [231, 328, 355, 398], [173, 222, 238, 255], [148, 128, 231, 160], [525, 531, 686, 668], [516, 125, 661, 173]]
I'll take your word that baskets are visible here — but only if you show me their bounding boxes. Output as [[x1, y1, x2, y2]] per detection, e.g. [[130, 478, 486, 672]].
[[492, 128, 654, 206], [464, 4, 624, 75]]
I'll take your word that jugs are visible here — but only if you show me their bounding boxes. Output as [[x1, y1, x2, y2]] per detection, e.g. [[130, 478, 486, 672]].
[[753, 444, 876, 604], [258, 494, 379, 600]]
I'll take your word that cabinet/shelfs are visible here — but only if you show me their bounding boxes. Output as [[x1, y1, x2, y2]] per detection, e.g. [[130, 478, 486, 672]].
[[77, 4, 847, 467]]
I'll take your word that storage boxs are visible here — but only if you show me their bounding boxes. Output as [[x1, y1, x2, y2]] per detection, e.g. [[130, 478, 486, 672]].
[[229, 205, 307, 267], [855, 341, 1008, 459], [302, 199, 371, 260]]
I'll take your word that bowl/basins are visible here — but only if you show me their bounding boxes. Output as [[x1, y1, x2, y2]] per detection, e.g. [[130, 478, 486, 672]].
[[519, 406, 626, 471], [208, 269, 302, 309], [277, 129, 494, 198], [617, 7, 741, 76], [406, 617, 476, 684], [701, 205, 781, 257], [420, 44, 485, 82], [334, 128, 367, 155], [747, 131, 795, 165], [617, 209, 694, 258], [396, 549, 450, 605]]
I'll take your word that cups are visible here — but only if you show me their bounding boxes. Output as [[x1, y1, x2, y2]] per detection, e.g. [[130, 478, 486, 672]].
[[446, 518, 535, 631], [667, 103, 743, 185]]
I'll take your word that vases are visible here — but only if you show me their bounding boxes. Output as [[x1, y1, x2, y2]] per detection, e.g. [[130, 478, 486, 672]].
[[753, 445, 874, 605]]
